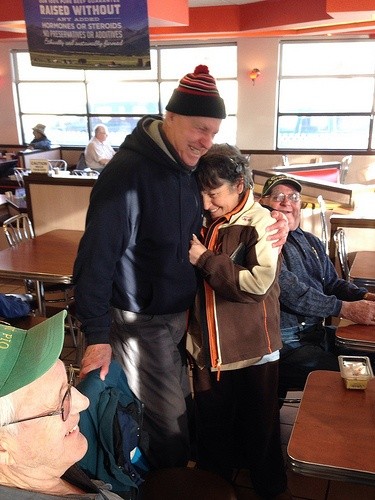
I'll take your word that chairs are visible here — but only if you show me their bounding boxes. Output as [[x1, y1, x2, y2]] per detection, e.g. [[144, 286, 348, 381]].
[[330, 228, 350, 283], [41, 287, 81, 347], [0, 160, 99, 190], [3, 214, 35, 293]]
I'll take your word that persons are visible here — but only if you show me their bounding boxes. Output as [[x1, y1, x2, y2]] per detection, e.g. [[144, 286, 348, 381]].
[[85, 124, 116, 171], [27, 123, 51, 152], [0, 310, 125, 500], [261, 175, 375, 378], [188, 144, 283, 458], [72, 64, 290, 472]]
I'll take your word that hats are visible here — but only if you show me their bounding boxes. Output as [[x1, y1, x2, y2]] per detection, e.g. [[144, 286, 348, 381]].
[[32, 124, 46, 137], [0, 310, 67, 397], [261, 174, 302, 197], [166, 65, 225, 119]]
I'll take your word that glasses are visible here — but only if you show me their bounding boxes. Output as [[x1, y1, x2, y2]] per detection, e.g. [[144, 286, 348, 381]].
[[264, 194, 301, 201], [2, 364, 74, 426]]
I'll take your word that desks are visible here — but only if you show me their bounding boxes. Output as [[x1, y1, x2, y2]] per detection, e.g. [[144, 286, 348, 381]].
[[345, 251, 375, 288], [335, 292, 375, 352], [287, 370, 375, 500], [1, 229, 85, 316], [4, 196, 28, 213]]
